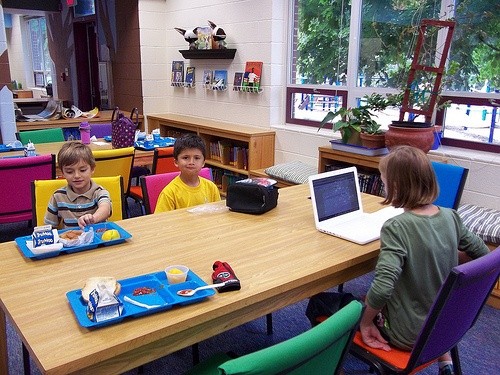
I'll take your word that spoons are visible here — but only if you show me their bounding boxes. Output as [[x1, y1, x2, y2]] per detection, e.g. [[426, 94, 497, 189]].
[[176, 283, 225, 296]]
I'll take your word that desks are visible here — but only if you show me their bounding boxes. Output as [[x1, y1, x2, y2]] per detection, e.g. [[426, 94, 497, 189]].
[[0, 139, 156, 156], [15, 109, 144, 132], [0, 183, 405, 375]]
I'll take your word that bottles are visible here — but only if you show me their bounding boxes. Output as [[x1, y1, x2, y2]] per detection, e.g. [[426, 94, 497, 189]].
[[79, 120, 90, 144]]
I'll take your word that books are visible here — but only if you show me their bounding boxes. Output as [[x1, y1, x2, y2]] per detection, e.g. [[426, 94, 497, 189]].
[[171, 61, 185, 87], [233, 61, 263, 91], [185, 67, 195, 87], [324, 164, 387, 199], [209, 139, 249, 192], [204, 70, 228, 90]]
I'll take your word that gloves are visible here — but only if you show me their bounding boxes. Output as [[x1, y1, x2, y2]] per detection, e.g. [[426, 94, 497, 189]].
[[212, 260, 240, 292]]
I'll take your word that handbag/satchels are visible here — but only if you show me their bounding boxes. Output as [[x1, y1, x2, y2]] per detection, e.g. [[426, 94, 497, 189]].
[[111, 106, 139, 149]]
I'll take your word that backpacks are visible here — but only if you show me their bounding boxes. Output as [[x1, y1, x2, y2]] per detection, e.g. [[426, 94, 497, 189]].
[[226, 177, 279, 215]]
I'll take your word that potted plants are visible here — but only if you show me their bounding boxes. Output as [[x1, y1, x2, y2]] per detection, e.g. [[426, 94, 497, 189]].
[[316, 13, 460, 155]]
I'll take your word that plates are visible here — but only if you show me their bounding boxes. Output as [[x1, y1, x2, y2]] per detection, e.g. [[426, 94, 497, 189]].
[[0, 144, 26, 151], [103, 134, 177, 151], [15, 221, 132, 259], [65, 268, 216, 329]]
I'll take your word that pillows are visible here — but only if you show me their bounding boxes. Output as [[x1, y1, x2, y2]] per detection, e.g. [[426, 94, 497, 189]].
[[458, 204, 499, 245], [265, 161, 316, 184]]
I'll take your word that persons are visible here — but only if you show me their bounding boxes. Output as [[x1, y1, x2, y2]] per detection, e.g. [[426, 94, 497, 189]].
[[153, 133, 221, 215], [305, 145, 491, 375], [43, 141, 112, 230]]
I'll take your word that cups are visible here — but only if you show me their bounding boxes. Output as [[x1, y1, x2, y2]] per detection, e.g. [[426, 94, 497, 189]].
[[165, 265, 189, 285]]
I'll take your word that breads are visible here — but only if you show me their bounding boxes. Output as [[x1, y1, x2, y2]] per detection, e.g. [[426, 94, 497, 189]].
[[82, 276, 121, 302]]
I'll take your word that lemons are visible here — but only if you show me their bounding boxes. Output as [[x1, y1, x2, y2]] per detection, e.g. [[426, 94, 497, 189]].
[[101, 230, 120, 241]]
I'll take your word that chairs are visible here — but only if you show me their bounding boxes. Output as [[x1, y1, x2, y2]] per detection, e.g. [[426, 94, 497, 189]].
[[31, 175, 125, 230], [89, 149, 135, 198], [130, 147, 175, 206], [140, 168, 214, 215], [319, 247, 500, 375], [0, 154, 56, 223], [88, 124, 112, 138], [15, 127, 64, 145], [215, 300, 367, 375], [429, 162, 469, 212]]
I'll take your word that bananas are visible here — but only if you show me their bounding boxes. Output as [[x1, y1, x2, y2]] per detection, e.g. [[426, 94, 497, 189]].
[[89, 107, 100, 118]]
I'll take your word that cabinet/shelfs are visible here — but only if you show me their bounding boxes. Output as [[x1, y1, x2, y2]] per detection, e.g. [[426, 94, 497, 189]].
[[147, 112, 275, 199], [317, 147, 387, 196]]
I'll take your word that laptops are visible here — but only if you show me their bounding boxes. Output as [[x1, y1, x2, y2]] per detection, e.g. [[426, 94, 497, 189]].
[[308, 166, 388, 244]]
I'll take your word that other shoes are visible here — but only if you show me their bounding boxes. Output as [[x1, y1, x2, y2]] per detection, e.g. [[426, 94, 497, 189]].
[[438, 363, 455, 375]]
